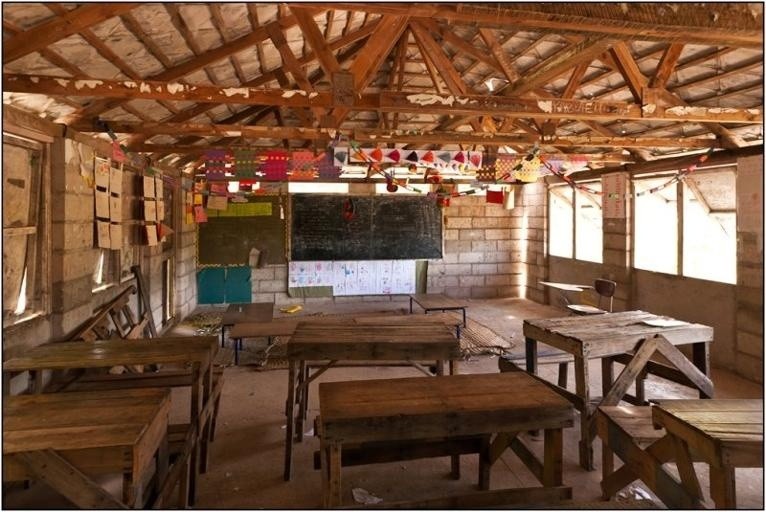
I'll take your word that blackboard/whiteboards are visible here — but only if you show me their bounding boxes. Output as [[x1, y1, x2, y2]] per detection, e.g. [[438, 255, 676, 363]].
[[287, 192, 445, 262]]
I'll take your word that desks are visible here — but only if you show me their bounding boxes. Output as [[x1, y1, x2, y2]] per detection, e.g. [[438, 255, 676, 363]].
[[540, 279, 594, 317], [410, 291, 467, 327]]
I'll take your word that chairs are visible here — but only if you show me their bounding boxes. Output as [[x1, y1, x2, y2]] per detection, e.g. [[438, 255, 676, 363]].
[[567, 277, 616, 315]]
